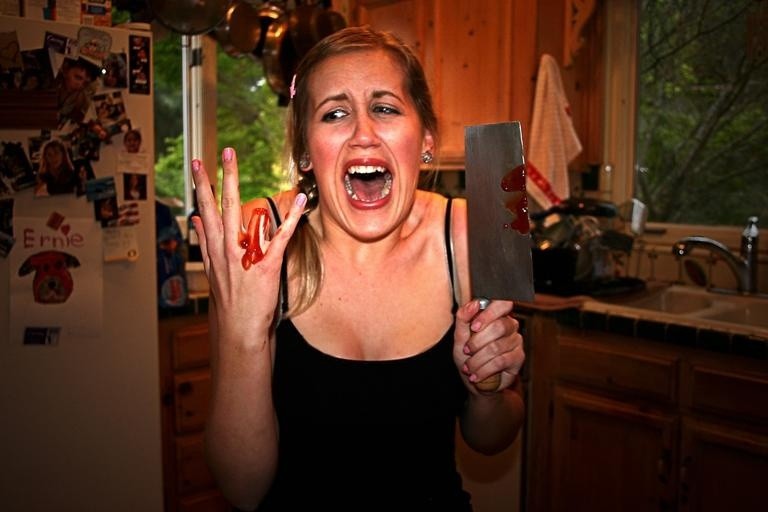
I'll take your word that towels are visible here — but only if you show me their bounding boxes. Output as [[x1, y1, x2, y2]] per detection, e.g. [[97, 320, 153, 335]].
[[526, 54, 583, 211]]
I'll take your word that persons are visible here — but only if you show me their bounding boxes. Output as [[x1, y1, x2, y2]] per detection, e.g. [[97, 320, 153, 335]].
[[35, 141, 76, 196], [125, 175, 142, 200], [50, 56, 99, 121], [182, 23, 539, 511], [123, 130, 143, 152]]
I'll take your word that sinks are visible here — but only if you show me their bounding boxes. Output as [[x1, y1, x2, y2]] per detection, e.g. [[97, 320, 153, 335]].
[[698, 297, 768, 333], [619, 284, 716, 318]]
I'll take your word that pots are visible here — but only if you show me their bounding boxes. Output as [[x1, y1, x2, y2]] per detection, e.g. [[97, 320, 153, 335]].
[[148, 1, 347, 108]]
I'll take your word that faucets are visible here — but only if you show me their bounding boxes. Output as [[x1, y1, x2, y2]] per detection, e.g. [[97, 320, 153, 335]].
[[672, 214, 760, 296]]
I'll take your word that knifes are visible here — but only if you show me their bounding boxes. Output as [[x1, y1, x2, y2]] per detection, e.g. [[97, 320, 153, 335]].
[[463, 120, 536, 395]]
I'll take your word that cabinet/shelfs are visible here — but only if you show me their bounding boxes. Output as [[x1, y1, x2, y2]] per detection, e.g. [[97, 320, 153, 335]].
[[161, 316, 223, 511], [527, 317, 767, 510]]
